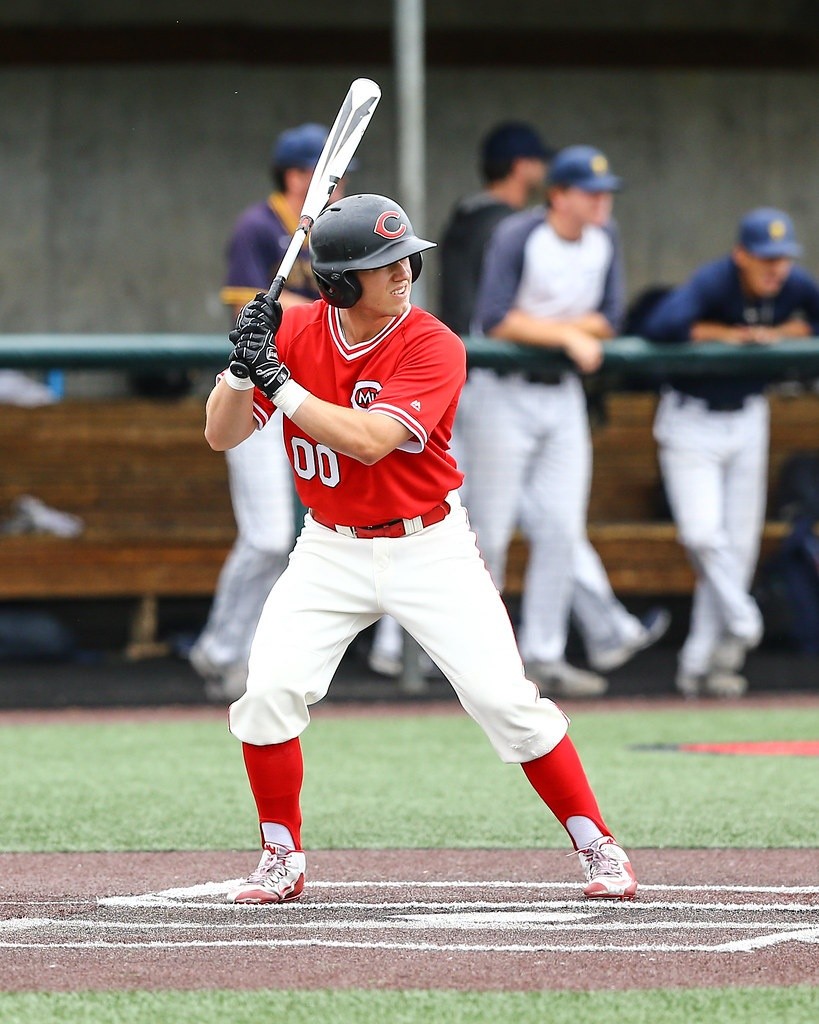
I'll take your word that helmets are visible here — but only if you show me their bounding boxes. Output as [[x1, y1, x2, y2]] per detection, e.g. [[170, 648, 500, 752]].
[[308, 193, 437, 309]]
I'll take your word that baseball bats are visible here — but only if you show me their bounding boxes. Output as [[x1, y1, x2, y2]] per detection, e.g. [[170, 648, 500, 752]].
[[228, 77, 384, 380]]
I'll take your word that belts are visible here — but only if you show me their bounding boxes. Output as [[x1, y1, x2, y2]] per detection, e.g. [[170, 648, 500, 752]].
[[311, 501, 451, 539]]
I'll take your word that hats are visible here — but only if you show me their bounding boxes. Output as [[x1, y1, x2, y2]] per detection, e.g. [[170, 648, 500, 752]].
[[546, 144, 619, 197], [737, 208, 802, 255], [272, 122, 357, 173], [484, 122, 555, 159]]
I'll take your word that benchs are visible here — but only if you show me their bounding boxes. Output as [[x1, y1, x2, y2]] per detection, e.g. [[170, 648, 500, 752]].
[[1, 385, 818, 658]]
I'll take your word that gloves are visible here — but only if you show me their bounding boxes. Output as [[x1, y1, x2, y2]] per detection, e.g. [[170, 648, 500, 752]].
[[236, 291, 283, 336], [228, 323, 291, 401]]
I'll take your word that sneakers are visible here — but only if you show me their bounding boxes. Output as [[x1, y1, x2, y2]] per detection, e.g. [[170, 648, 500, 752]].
[[677, 635, 749, 699], [226, 842, 305, 902], [567, 834, 637, 899], [524, 660, 608, 698], [590, 609, 672, 674]]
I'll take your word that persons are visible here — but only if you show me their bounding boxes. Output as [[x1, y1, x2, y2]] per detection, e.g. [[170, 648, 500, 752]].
[[204, 193, 638, 903], [368, 120, 670, 679], [187, 122, 360, 702], [643, 207, 819, 695], [471, 145, 628, 697]]
